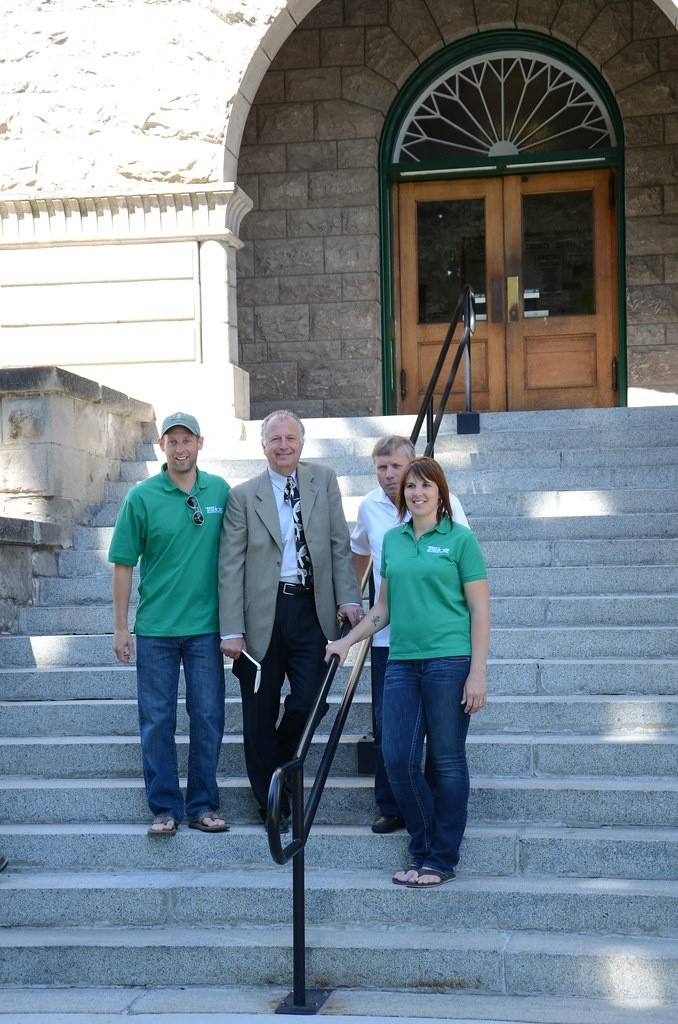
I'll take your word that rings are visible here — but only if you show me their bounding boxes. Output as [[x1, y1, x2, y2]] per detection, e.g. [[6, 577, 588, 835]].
[[359, 615, 364, 618]]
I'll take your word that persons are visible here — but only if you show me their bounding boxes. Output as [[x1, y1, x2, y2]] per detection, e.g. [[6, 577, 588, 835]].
[[108, 410, 232, 836], [349, 435, 471, 833], [325, 457, 491, 890], [217, 409, 367, 834]]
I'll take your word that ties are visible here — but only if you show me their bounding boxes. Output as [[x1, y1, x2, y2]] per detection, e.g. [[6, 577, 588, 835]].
[[286, 477, 313, 588]]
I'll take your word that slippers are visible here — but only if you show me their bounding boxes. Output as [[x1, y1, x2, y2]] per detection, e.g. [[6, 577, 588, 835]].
[[392, 865, 455, 888]]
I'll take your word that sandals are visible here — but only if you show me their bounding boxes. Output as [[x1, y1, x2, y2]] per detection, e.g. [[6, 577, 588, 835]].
[[190, 813, 230, 832], [149, 816, 176, 836]]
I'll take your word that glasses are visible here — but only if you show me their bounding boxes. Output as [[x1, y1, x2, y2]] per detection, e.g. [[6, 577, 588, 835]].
[[185, 495, 204, 525]]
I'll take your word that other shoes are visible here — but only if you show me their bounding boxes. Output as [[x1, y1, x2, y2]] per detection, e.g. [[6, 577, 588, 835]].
[[371, 815, 406, 833]]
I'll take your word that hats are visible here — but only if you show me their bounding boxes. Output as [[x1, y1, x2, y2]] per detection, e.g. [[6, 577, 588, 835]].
[[162, 412, 201, 438]]
